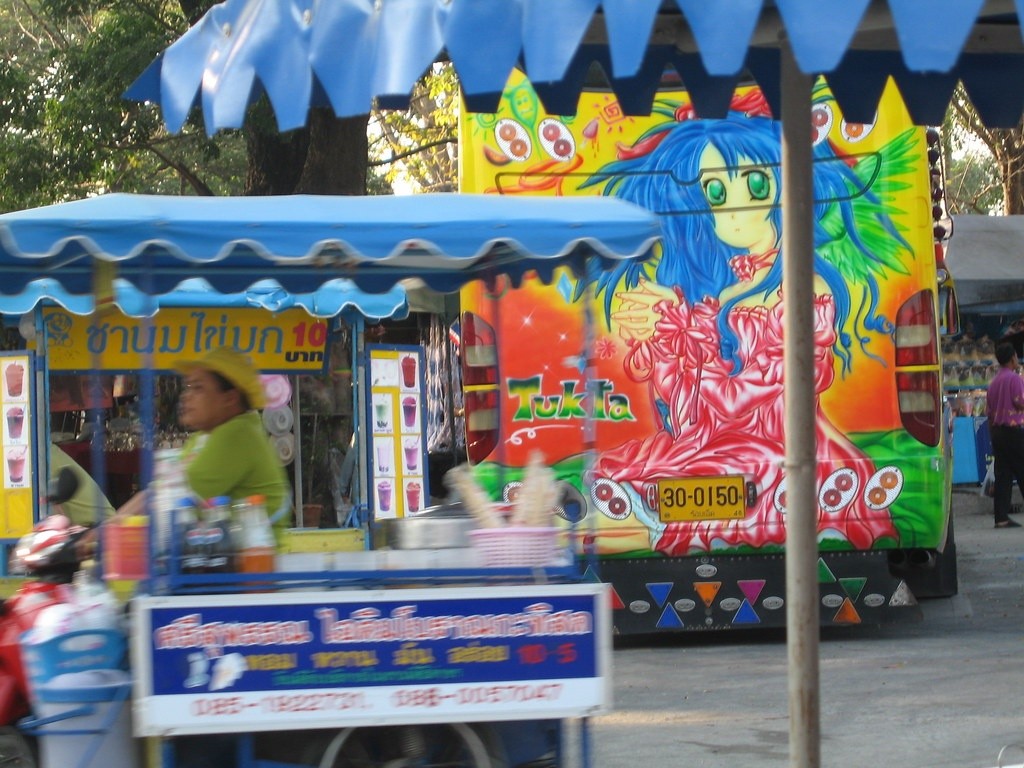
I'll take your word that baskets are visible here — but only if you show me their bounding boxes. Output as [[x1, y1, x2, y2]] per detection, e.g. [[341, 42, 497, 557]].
[[18, 625, 125, 718]]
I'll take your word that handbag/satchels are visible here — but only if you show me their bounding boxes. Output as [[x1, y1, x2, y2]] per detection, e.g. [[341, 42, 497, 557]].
[[980, 456, 995, 498]]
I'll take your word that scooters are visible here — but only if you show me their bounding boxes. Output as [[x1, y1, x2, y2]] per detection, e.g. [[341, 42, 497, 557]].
[[0, 465, 126, 768]]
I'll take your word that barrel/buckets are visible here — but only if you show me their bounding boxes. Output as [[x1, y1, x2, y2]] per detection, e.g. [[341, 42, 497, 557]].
[[20, 669, 140, 768], [466, 526, 560, 586]]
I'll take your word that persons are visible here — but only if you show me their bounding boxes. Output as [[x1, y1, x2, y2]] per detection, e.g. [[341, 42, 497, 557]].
[[73, 343, 292, 571], [50, 441, 114, 529], [987, 341, 1024, 529]]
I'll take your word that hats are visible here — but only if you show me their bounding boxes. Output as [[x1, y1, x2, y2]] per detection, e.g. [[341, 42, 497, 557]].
[[173, 345, 266, 409]]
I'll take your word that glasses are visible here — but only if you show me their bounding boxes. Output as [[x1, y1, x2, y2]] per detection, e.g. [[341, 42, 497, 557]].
[[184, 381, 220, 395]]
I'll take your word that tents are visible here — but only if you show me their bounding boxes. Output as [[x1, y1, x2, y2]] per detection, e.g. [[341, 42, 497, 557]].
[[0, 192, 664, 588]]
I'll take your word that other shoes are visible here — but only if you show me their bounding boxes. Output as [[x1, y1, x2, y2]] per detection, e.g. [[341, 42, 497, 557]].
[[994, 518, 1021, 528]]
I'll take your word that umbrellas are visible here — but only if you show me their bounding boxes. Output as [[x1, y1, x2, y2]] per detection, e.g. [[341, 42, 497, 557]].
[[123, 0, 1024, 768]]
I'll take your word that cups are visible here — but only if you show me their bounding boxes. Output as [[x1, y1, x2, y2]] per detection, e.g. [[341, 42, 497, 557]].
[[406, 488, 420, 512], [150, 449, 192, 595], [377, 447, 389, 472], [5, 370, 23, 396], [405, 446, 417, 470], [7, 458, 24, 483], [375, 403, 388, 428], [401, 363, 415, 388], [7, 414, 24, 438], [403, 404, 416, 431], [377, 487, 391, 511]]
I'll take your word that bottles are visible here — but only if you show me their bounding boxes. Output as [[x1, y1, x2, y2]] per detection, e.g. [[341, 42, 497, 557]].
[[178, 495, 278, 596]]
